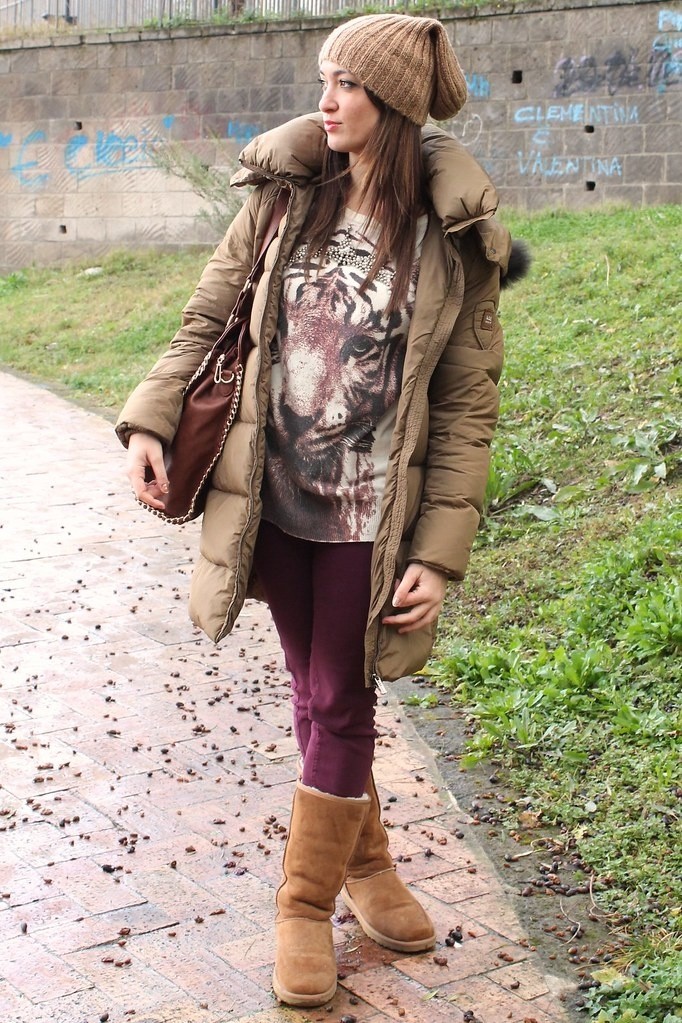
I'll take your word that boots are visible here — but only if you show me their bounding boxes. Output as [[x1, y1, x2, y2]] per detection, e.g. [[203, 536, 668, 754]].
[[340, 767, 437, 953], [270, 775, 372, 1006]]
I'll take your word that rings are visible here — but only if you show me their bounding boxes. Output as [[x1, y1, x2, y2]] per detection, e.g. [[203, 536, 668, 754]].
[[131, 487, 136, 494]]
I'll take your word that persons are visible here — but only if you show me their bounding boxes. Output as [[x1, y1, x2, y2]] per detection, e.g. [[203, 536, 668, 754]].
[[110, 15, 534, 1012]]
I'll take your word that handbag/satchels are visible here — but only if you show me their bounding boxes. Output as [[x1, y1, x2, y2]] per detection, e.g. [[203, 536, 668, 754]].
[[137, 315, 251, 525]]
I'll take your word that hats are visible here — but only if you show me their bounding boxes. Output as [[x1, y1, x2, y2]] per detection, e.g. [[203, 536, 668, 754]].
[[319, 12, 468, 128]]
